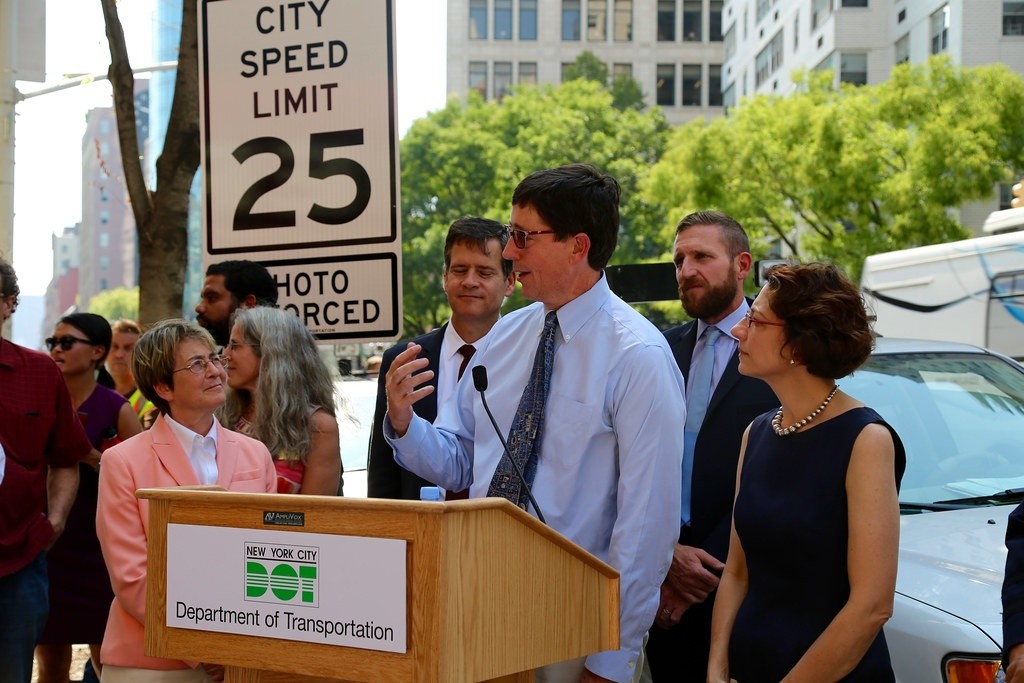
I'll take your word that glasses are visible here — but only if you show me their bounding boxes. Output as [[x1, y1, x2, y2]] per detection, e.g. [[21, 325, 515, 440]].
[[744, 311, 783, 332], [227, 341, 260, 352], [169, 354, 228, 374], [45, 334, 93, 351], [504, 224, 561, 248]]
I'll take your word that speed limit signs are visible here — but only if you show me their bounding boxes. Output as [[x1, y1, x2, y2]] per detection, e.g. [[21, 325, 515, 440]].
[[197, 0, 402, 343]]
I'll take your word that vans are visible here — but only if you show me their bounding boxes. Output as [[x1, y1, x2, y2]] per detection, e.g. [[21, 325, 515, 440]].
[[855, 208, 1023, 368]]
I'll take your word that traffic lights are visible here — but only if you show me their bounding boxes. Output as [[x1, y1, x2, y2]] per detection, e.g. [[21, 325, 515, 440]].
[[1010, 180, 1024, 209]]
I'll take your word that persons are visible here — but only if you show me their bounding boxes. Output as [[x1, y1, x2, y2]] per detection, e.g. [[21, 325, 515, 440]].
[[222, 306, 344, 496], [707, 261, 906, 682], [0, 254, 91, 683], [36, 313, 144, 683], [367, 216, 515, 501], [195, 260, 278, 347], [645, 211, 783, 683], [1001, 501, 1024, 683], [383, 163, 687, 683], [101, 318, 157, 422], [95, 319, 276, 683]]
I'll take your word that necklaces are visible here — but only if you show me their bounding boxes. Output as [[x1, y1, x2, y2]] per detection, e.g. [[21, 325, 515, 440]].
[[772, 385, 840, 436]]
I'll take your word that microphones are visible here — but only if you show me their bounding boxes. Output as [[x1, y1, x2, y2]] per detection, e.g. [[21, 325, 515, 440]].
[[472, 365, 547, 524]]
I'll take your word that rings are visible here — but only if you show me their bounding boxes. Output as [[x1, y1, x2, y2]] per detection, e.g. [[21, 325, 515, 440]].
[[663, 609, 671, 614]]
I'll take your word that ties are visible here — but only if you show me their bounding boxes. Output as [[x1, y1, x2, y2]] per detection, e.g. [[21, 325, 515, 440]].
[[487, 311, 563, 511], [680, 328, 726, 525], [445, 345, 477, 501]]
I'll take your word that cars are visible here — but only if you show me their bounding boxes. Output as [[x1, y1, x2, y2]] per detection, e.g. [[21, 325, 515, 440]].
[[837, 338, 1023, 683], [333, 375, 378, 497]]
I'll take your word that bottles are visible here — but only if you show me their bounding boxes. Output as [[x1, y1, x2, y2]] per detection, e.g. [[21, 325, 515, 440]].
[[419, 486, 440, 502]]
